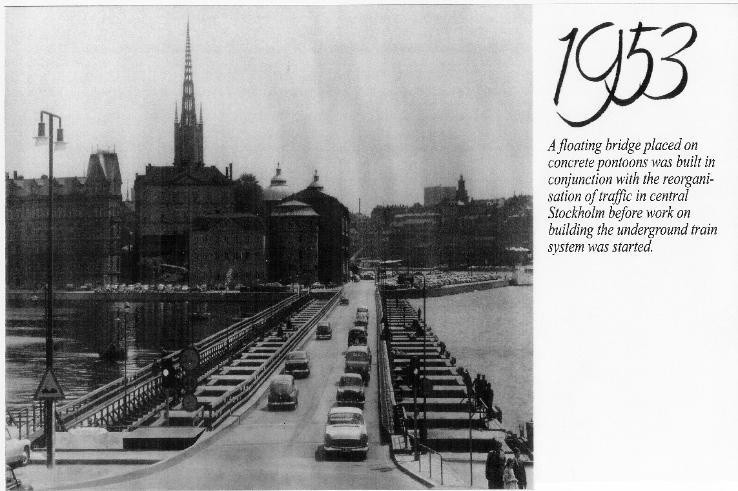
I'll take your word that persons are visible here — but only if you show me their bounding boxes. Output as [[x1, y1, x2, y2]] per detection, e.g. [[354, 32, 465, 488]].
[[463, 372, 494, 420], [485, 439, 506, 488], [410, 307, 425, 336], [286, 318, 293, 330], [502, 458, 518, 489], [418, 419, 428, 455], [512, 447, 528, 489], [277, 324, 284, 338]]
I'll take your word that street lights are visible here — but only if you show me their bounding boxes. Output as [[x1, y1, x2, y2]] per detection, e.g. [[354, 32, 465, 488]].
[[33, 111, 67, 369]]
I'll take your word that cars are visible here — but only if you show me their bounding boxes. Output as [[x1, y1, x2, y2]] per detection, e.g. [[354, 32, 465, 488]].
[[266, 351, 311, 410], [315, 320, 332, 340], [339, 294, 349, 305], [235, 280, 286, 291], [5, 425, 31, 491], [323, 306, 372, 455]]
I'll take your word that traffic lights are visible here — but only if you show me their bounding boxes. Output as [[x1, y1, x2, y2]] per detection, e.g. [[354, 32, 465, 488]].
[[162, 365, 173, 384], [410, 356, 420, 385]]
